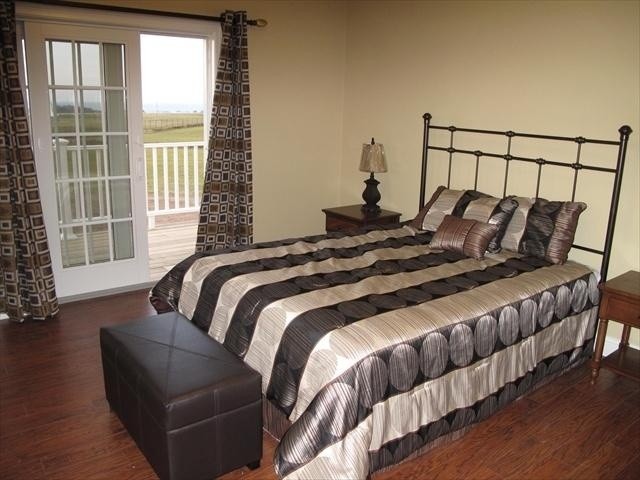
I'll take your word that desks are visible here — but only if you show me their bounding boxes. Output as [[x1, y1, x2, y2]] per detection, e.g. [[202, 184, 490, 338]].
[[590, 270, 640, 384]]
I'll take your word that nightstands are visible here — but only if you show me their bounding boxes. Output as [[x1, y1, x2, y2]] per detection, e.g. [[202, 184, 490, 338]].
[[321, 204, 402, 239]]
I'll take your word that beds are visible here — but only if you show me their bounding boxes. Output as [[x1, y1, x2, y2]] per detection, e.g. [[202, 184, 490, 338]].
[[147, 113, 633, 480]]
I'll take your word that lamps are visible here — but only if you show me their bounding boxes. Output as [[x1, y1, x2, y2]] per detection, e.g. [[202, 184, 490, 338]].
[[359, 137, 389, 216]]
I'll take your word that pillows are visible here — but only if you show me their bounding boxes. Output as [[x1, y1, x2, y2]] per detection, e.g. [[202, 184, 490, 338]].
[[408, 186, 587, 266]]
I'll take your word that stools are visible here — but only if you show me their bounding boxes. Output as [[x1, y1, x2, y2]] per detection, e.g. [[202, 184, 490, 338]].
[[99, 310, 265, 480]]
